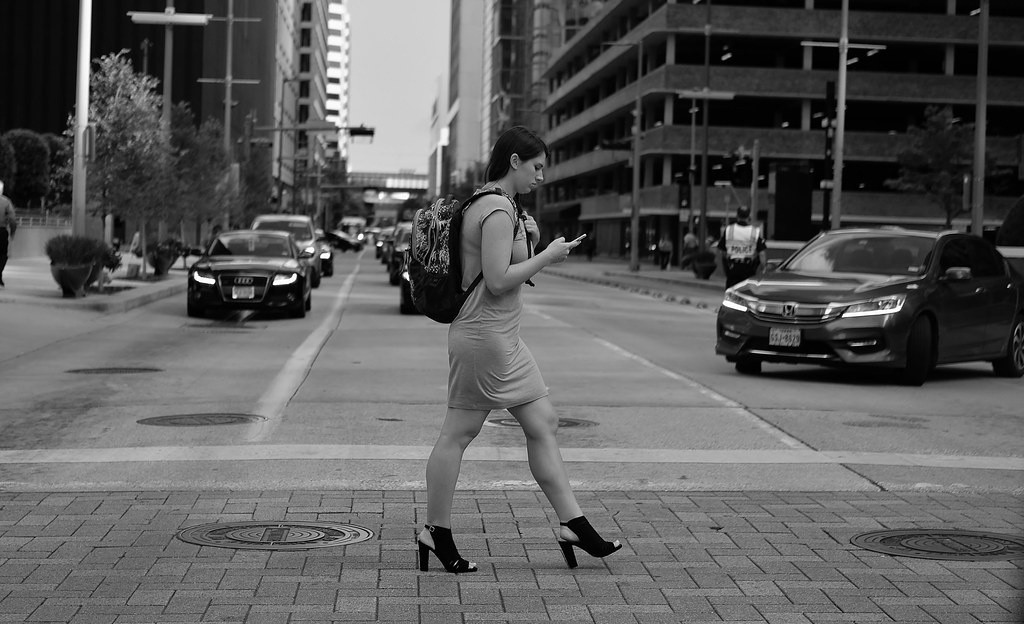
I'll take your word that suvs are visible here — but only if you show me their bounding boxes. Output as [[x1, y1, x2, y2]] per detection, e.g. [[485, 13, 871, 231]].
[[248, 214, 324, 287]]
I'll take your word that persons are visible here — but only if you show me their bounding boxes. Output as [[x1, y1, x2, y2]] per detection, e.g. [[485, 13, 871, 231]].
[[659, 232, 712, 270], [0, 182, 17, 286], [586, 234, 594, 261], [717, 206, 767, 291], [417, 127, 623, 573]]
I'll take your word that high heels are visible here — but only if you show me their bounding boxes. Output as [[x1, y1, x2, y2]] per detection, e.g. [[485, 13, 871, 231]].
[[416, 524, 478, 573], [558, 515, 623, 570]]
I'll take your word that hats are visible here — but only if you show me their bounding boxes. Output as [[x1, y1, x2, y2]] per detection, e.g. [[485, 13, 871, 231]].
[[737, 206, 751, 219]]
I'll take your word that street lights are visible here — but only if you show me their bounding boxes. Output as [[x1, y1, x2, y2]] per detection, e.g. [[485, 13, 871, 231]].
[[675, 89, 735, 250], [276, 72, 313, 212], [126, 2, 213, 277]]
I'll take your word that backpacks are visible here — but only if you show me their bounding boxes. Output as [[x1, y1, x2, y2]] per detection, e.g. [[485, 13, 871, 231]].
[[405, 189, 534, 324]]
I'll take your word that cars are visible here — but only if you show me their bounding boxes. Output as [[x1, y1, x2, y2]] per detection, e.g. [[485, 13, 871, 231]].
[[327, 213, 423, 316], [184, 229, 314, 319], [715, 228, 1024, 386], [319, 241, 336, 277]]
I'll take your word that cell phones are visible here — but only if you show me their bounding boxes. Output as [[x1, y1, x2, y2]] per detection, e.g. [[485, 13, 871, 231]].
[[572, 234, 587, 242]]
